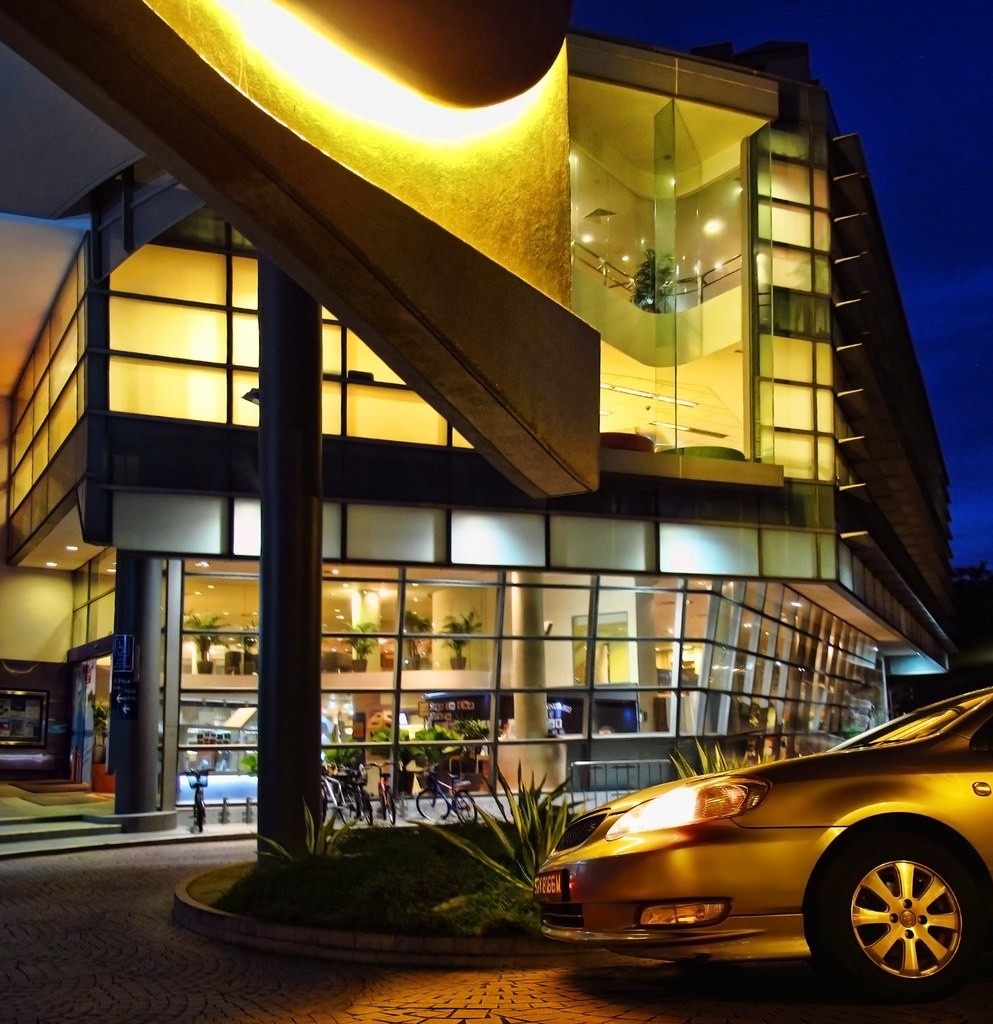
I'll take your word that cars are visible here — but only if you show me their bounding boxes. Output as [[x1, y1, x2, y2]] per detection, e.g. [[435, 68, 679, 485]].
[[535, 687, 993, 986]]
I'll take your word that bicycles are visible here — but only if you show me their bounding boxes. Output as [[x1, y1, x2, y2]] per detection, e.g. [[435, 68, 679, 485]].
[[368, 761, 399, 826], [415, 760, 477, 822], [181, 769, 216, 832], [321, 759, 373, 826]]
